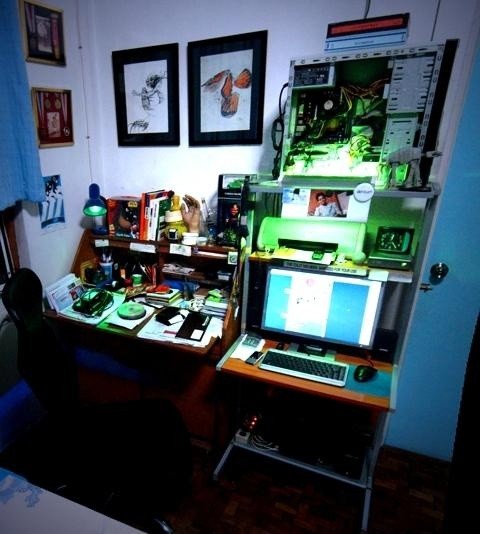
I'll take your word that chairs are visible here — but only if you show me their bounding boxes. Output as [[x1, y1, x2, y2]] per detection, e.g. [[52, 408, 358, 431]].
[[1, 265, 200, 533]]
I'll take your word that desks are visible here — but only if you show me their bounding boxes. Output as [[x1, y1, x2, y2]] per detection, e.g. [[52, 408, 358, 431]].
[[41, 198, 257, 464]]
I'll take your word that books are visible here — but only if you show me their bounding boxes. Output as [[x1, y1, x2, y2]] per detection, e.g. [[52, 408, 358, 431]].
[[139, 189, 172, 243], [144, 286, 230, 319]]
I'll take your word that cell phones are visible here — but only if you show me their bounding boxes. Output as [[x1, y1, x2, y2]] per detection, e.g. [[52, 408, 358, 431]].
[[245, 351, 264, 365], [313, 246, 325, 260]]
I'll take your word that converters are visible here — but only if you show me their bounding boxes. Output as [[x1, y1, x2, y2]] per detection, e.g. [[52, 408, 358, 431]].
[[82, 288, 104, 303]]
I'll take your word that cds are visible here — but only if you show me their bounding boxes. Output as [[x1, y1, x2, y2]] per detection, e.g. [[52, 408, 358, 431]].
[[117, 301, 147, 320]]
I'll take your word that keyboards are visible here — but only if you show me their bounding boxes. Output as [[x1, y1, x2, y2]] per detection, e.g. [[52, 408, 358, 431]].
[[258, 350, 349, 387]]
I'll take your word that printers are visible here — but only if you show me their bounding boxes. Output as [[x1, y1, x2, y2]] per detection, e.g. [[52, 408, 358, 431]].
[[256, 215, 367, 260]]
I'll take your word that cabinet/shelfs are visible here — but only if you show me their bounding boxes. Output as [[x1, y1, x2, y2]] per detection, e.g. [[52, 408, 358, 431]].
[[211, 181, 447, 534]]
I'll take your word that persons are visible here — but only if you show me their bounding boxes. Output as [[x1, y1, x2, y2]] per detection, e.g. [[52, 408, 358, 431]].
[[313, 191, 344, 218], [180, 193, 202, 234]]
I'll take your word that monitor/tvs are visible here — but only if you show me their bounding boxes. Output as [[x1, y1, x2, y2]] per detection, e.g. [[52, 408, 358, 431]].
[[260, 267, 385, 356]]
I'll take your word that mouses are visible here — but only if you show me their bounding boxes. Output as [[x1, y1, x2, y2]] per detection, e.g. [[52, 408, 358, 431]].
[[354, 364, 378, 381]]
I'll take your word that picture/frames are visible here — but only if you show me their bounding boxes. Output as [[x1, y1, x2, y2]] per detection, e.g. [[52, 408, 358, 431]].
[[18, 0, 67, 68], [187, 28, 269, 147], [110, 40, 181, 146], [31, 85, 76, 149]]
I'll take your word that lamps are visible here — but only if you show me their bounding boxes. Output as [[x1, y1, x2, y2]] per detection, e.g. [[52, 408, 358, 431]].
[[83, 181, 110, 235]]
